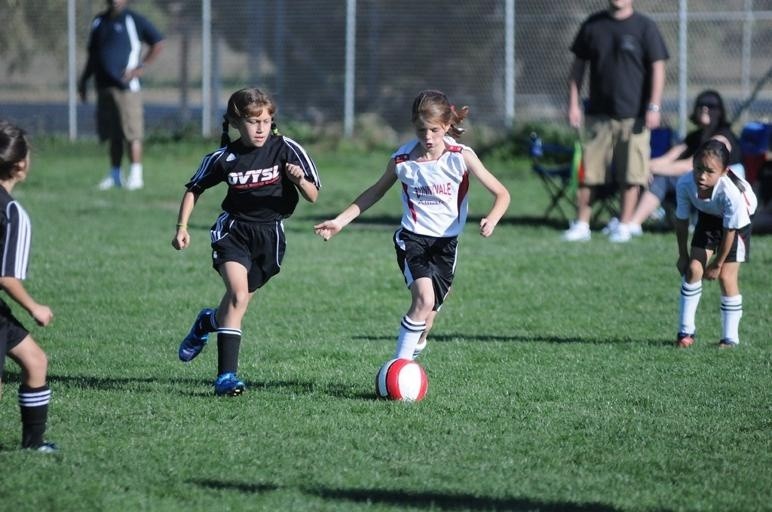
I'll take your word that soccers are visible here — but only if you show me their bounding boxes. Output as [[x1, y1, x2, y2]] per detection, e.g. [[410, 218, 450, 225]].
[[375, 359, 428, 401]]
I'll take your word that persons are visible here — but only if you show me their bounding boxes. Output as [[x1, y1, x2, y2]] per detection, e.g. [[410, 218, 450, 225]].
[[315, 90, 511, 360], [171, 87, 322, 396], [0, 122, 60, 455], [676, 139, 753, 350], [599, 91, 748, 238], [80, 0, 166, 192], [561, 0, 669, 243]]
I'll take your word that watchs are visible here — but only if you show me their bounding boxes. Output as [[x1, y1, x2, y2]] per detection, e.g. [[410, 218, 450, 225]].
[[645, 104, 660, 115]]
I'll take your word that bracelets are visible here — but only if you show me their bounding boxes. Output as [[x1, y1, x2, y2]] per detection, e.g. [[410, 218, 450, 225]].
[[177, 223, 190, 230], [137, 63, 153, 74]]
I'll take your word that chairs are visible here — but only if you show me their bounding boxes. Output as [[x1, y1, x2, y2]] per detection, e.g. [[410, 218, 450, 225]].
[[527, 132, 579, 228], [741, 120, 772, 234], [595, 124, 675, 233]]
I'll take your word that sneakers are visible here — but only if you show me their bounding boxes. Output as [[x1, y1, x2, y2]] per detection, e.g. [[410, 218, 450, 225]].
[[561, 220, 591, 241], [215, 368, 244, 398], [127, 173, 143, 190], [719, 339, 737, 349], [99, 176, 121, 189], [603, 217, 642, 243], [178, 309, 216, 362], [676, 333, 695, 348], [22, 443, 59, 452]]
